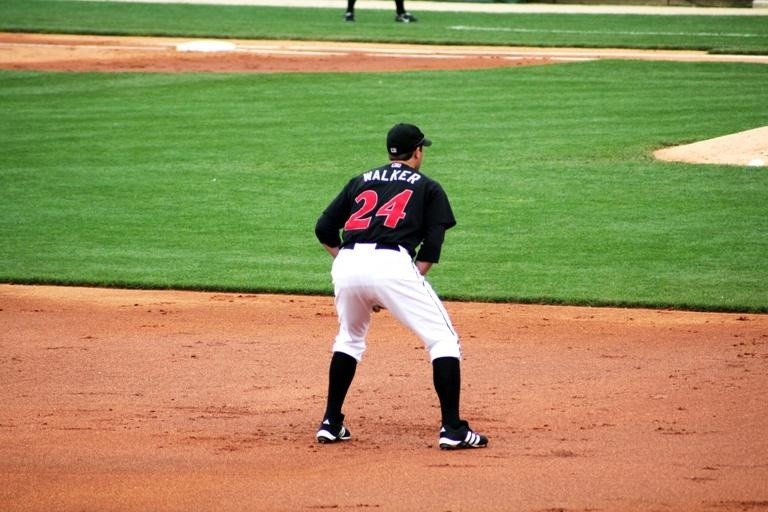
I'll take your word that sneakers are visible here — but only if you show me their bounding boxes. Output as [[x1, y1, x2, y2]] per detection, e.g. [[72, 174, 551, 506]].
[[439, 420, 488, 449], [316, 413, 351, 444], [343, 12, 354, 22], [396, 12, 417, 22]]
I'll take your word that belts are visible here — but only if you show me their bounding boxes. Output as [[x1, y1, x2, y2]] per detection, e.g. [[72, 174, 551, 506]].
[[344, 242, 399, 250]]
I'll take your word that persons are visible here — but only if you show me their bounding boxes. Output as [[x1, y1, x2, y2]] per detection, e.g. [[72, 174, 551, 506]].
[[341, 0, 418, 23], [316, 122, 488, 450]]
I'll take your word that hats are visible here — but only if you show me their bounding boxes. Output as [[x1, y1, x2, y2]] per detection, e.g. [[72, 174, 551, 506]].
[[387, 123, 432, 156]]
